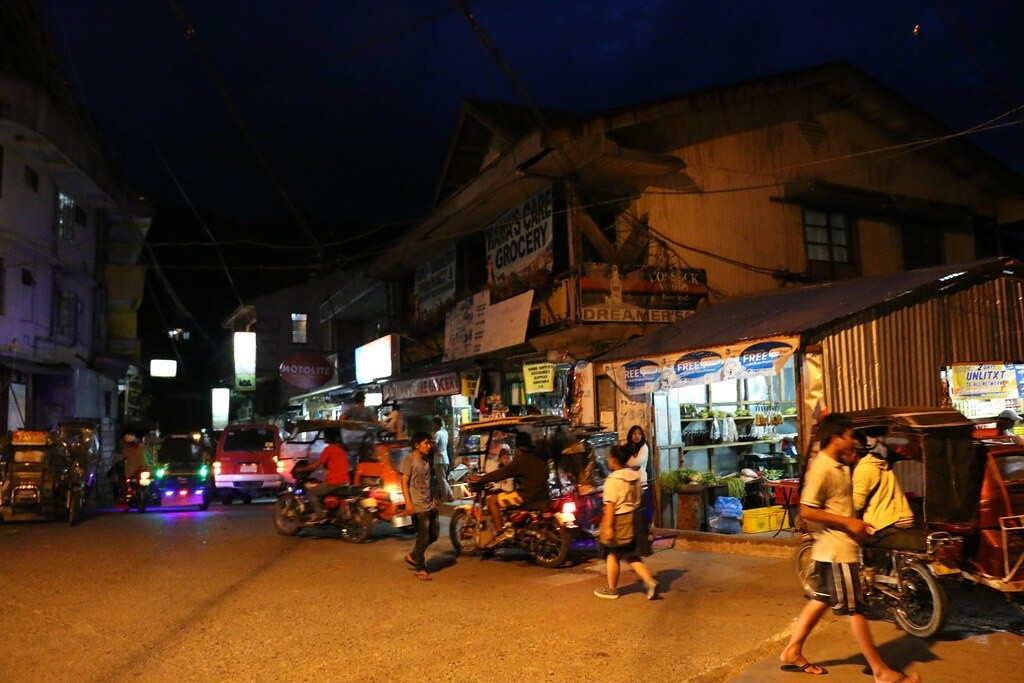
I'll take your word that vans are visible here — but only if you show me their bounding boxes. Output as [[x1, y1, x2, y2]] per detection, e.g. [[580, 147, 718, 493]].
[[212, 419, 286, 505], [152, 432, 217, 510]]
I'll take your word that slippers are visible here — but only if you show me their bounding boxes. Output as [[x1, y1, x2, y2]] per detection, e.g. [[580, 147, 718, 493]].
[[413, 567, 432, 581], [880, 674, 916, 683], [405, 554, 428, 570], [780, 660, 828, 674]]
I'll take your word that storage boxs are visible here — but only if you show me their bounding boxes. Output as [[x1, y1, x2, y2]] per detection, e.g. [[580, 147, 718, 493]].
[[774, 482, 800, 505], [759, 506, 790, 531], [740, 510, 773, 533]]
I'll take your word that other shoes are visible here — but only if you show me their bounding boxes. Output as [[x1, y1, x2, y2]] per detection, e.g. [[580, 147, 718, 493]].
[[304, 514, 328, 525], [594, 584, 620, 599], [648, 579, 660, 600]]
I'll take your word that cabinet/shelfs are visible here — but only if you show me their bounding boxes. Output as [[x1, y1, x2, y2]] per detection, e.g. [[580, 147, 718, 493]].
[[672, 398, 801, 531]]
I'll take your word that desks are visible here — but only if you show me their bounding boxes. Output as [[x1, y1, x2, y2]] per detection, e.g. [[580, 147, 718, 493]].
[[763, 483, 801, 538]]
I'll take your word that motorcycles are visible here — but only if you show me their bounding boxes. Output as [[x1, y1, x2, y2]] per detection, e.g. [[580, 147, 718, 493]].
[[106, 448, 153, 514]]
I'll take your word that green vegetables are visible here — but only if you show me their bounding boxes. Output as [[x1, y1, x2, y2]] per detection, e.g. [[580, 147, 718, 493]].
[[659, 467, 748, 501], [697, 408, 752, 419]]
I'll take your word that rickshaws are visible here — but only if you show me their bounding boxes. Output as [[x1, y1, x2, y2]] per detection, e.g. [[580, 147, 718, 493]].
[[792, 403, 1024, 642], [443, 413, 624, 568], [0, 412, 104, 527], [270, 413, 446, 546]]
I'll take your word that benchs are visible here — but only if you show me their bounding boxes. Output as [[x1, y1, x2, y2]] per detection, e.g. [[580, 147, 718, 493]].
[[934, 517, 980, 538]]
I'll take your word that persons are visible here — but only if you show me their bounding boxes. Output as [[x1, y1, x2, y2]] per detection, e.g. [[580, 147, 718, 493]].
[[198, 429, 218, 510], [993, 410, 1023, 443], [840, 429, 915, 546], [383, 404, 405, 437], [293, 426, 351, 526], [594, 445, 661, 600], [622, 425, 655, 557], [482, 449, 514, 509], [780, 412, 921, 683], [469, 431, 553, 546], [107, 432, 152, 503], [485, 440, 513, 473], [429, 416, 454, 506], [339, 390, 378, 422], [398, 431, 441, 581]]
